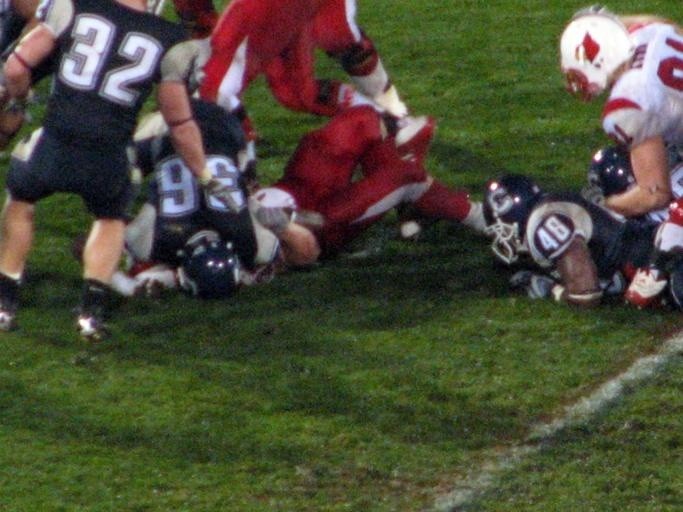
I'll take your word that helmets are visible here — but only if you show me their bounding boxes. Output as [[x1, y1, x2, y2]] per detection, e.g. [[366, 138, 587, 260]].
[[481, 174, 549, 265], [179, 226, 244, 296], [587, 145, 637, 194], [560, 11, 638, 103]]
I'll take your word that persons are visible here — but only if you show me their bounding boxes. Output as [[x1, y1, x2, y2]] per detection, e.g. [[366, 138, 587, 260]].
[[2, 1, 487, 341], [560, 2, 683, 309], [483, 174, 683, 310]]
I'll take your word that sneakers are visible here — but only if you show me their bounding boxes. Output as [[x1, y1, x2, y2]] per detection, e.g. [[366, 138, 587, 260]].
[[625, 266, 670, 310], [1, 302, 21, 333], [77, 311, 110, 342], [374, 86, 472, 238]]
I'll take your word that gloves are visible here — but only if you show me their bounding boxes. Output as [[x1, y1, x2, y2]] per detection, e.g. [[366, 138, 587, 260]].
[[202, 169, 244, 210], [512, 269, 562, 300], [255, 202, 289, 236], [133, 265, 174, 298]]
